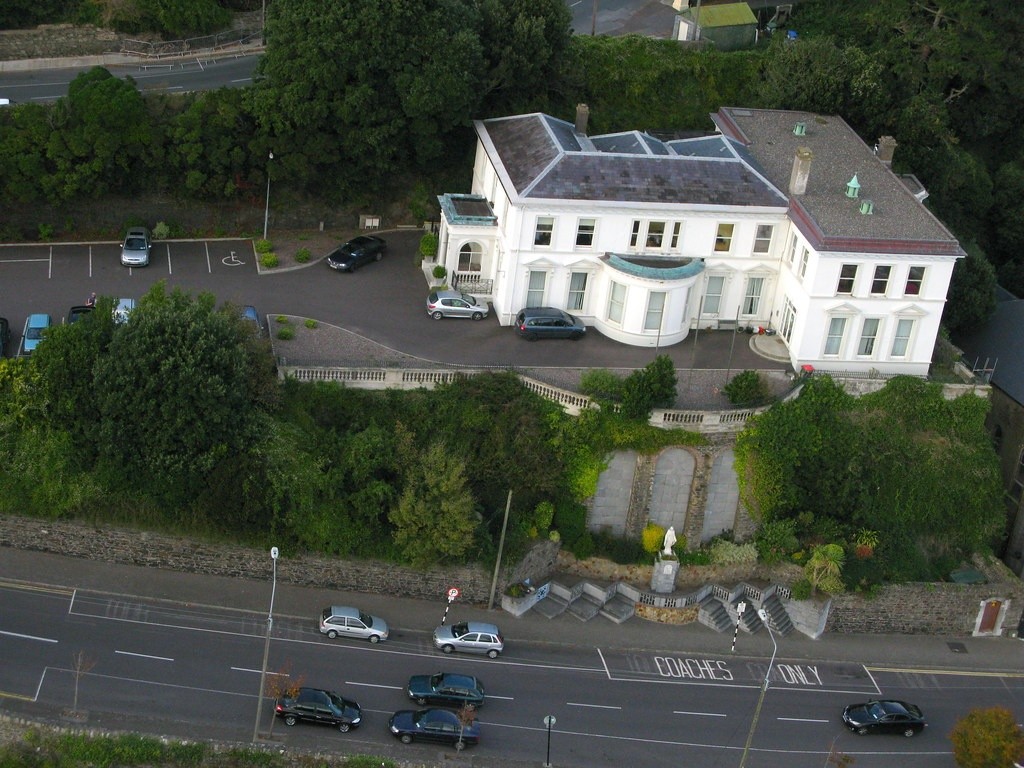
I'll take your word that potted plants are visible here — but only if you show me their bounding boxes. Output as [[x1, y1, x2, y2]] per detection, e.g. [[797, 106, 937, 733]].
[[432, 265, 447, 284], [419, 232, 439, 263]]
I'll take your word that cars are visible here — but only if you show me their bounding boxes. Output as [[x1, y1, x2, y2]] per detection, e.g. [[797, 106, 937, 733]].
[[319, 606, 389, 644], [425, 289, 490, 320], [110, 298, 137, 326], [842, 697, 928, 737], [514, 306, 587, 342], [433, 621, 505, 660], [387, 708, 481, 750], [237, 304, 265, 341], [15, 312, 53, 359], [66, 304, 97, 326], [118, 226, 152, 268], [406, 670, 485, 712], [326, 235, 388, 273], [272, 687, 361, 733], [0, 318, 12, 359]]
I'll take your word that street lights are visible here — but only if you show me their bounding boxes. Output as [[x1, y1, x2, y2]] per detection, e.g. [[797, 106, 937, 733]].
[[261, 148, 275, 242], [253, 547, 280, 744], [741, 607, 778, 768]]
